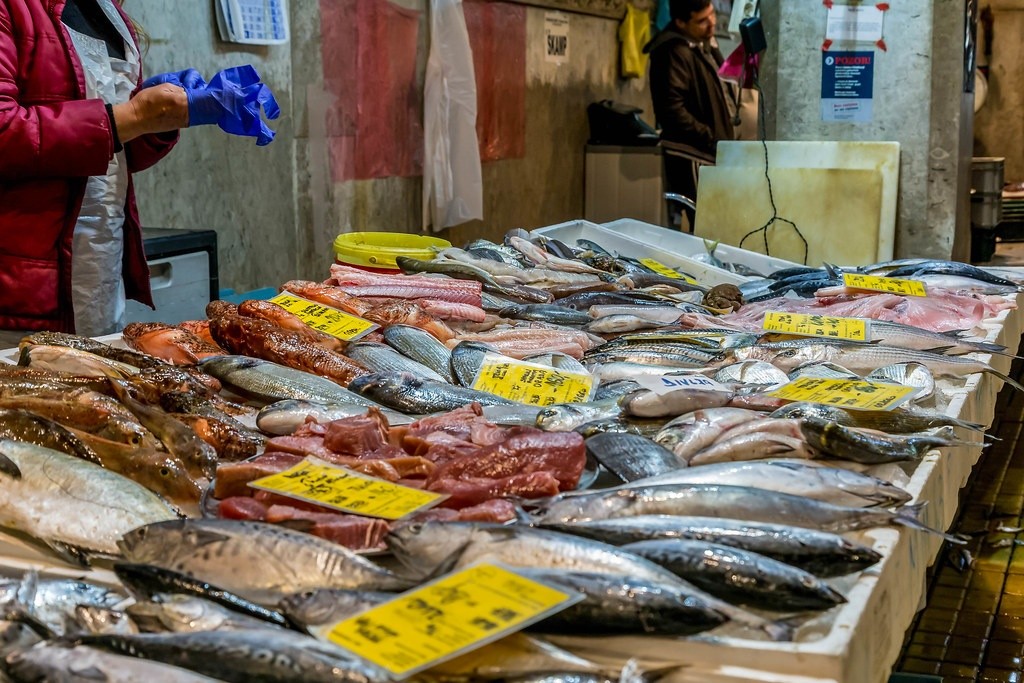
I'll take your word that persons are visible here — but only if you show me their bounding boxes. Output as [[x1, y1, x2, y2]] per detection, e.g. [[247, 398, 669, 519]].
[[642, 0, 734, 233], [0, 0, 245, 350]]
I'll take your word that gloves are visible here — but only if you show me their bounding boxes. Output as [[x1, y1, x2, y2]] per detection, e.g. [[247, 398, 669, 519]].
[[139, 63, 281, 146]]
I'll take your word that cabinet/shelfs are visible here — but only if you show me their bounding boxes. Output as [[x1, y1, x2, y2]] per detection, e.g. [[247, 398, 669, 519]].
[[124, 226, 219, 327]]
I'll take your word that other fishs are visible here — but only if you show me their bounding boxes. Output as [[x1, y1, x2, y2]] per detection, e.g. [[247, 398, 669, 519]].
[[0, 229, 1024, 683]]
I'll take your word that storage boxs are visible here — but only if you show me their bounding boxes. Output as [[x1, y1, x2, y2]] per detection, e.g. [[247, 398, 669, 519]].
[[529, 219, 1024, 683]]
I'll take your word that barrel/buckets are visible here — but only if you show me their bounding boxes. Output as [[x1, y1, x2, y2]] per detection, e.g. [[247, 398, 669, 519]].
[[333, 232, 452, 274]]
